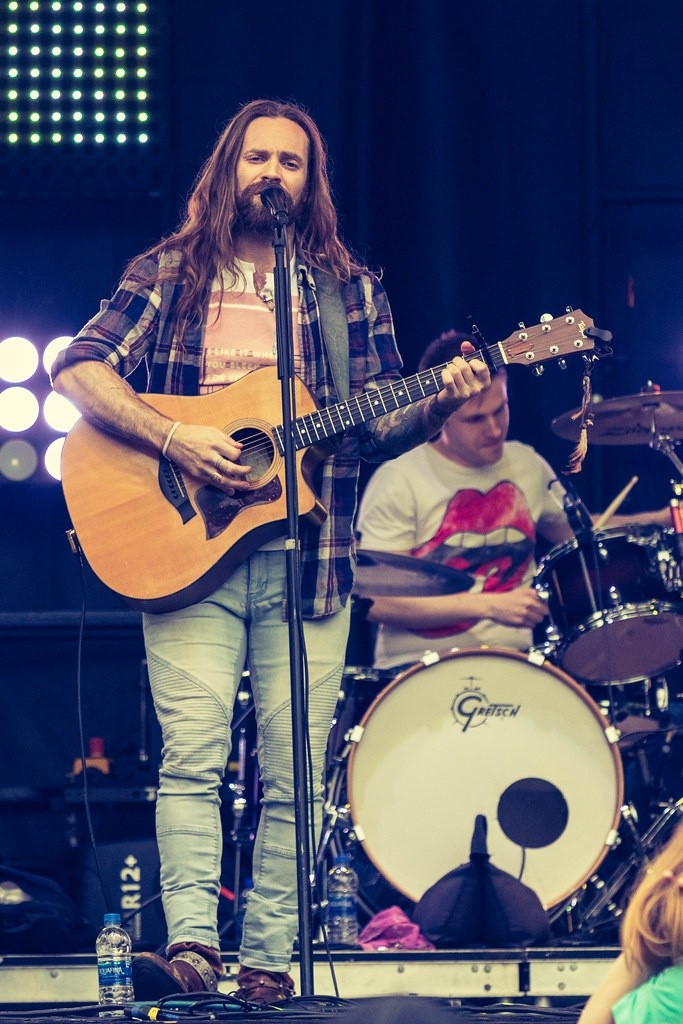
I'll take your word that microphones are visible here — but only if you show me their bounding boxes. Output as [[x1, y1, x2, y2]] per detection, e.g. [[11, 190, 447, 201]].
[[260, 183, 288, 227]]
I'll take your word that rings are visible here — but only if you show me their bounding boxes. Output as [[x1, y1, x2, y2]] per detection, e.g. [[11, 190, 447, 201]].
[[216, 457, 224, 468], [210, 470, 224, 485]]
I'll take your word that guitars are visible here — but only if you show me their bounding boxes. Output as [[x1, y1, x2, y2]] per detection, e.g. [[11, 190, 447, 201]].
[[60, 307, 613, 615]]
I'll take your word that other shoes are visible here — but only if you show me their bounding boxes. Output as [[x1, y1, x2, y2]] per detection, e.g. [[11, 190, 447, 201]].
[[235, 964, 297, 1006], [132, 941, 224, 1001]]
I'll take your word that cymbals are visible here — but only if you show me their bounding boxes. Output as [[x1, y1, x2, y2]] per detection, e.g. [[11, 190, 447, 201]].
[[551, 389, 683, 446], [349, 542, 478, 600]]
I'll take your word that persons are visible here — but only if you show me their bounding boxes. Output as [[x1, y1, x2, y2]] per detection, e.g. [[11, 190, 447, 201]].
[[575, 816, 683, 1023], [353, 329, 683, 672], [49, 97, 491, 1000]]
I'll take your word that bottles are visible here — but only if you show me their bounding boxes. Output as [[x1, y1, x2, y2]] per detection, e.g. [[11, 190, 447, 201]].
[[96, 912, 138, 1019], [325, 855, 360, 946]]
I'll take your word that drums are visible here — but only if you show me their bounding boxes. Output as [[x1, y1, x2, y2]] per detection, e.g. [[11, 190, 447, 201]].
[[346, 645, 626, 914], [530, 522, 683, 683]]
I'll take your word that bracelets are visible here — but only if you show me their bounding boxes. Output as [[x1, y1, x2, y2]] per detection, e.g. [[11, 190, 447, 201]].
[[162, 423, 183, 462]]
